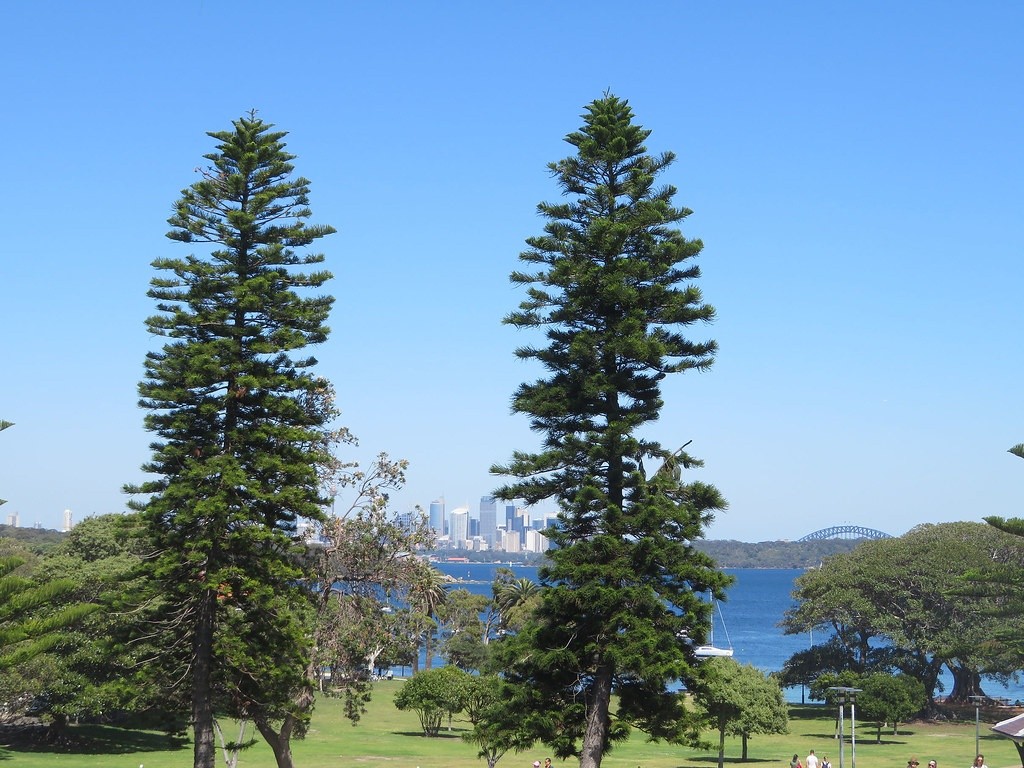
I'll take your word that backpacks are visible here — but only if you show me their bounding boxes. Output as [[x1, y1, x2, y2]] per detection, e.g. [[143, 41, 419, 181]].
[[822, 762, 829, 768]]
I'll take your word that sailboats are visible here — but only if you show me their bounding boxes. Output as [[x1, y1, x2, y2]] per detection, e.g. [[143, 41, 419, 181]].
[[685, 588, 735, 656]]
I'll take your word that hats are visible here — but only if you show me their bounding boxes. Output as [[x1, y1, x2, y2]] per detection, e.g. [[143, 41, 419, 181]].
[[908, 757, 919, 765], [532, 761, 540, 767]]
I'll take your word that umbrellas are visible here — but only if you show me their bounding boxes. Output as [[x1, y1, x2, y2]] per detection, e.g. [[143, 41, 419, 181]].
[[989, 713, 1024, 743]]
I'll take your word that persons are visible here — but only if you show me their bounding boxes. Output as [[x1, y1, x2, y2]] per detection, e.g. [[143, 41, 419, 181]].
[[805, 750, 819, 768], [821, 756, 831, 768], [533, 758, 554, 768], [378, 668, 382, 679], [907, 757, 919, 768], [928, 760, 937, 768], [790, 754, 802, 768], [970, 755, 988, 768]]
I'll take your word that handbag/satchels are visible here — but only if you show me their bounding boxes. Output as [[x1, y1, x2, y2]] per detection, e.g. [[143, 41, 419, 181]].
[[797, 763, 802, 768]]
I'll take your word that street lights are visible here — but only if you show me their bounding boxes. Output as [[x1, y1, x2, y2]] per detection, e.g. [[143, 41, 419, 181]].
[[844, 688, 864, 768], [968, 695, 986, 758], [829, 686, 854, 768]]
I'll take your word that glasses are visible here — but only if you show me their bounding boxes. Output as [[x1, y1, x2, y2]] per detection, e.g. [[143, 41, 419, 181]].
[[929, 764, 934, 767]]
